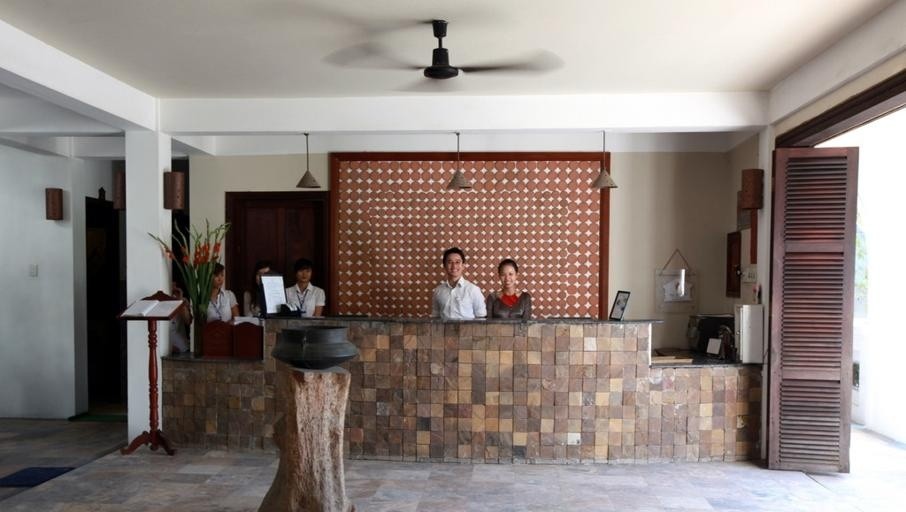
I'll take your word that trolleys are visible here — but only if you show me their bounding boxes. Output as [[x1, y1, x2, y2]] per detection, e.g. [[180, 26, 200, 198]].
[[324, 20, 564, 91]]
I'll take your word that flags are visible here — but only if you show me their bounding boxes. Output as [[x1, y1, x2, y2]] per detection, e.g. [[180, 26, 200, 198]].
[[193, 317, 206, 356]]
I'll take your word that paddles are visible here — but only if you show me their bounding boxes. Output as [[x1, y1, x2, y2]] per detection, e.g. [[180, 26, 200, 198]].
[[0, 465, 75, 488]]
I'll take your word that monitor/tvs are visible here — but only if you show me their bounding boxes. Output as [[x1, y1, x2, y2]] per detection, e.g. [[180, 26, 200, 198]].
[[120, 299, 184, 318]]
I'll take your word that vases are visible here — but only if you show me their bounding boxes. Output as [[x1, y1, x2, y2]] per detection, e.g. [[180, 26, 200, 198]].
[[148, 218, 231, 325]]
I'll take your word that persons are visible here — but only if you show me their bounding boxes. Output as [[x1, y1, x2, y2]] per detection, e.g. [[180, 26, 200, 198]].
[[429, 246, 488, 321], [486, 258, 532, 319], [206, 262, 240, 325], [284, 258, 327, 316], [242, 260, 274, 317], [171, 276, 194, 354]]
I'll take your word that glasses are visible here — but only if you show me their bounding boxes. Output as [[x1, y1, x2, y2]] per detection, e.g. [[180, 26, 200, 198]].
[[164, 172, 186, 211], [446, 132, 472, 189], [45, 187, 63, 220], [740, 168, 763, 210], [296, 134, 320, 188], [112, 170, 125, 211], [591, 131, 618, 189]]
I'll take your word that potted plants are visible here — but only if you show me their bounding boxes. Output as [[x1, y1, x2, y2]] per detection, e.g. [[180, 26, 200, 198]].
[[609, 290, 631, 321], [654, 268, 700, 314], [726, 230, 742, 299]]
